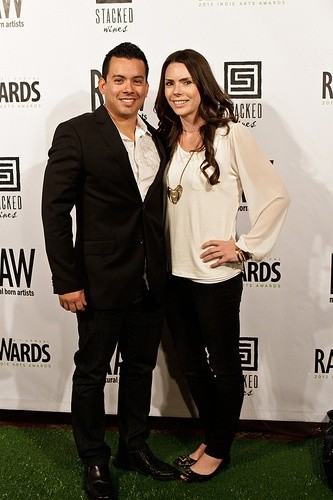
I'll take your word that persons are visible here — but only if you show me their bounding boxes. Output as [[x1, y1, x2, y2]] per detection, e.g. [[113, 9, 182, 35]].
[[154, 49, 291, 483], [41, 43, 180, 500]]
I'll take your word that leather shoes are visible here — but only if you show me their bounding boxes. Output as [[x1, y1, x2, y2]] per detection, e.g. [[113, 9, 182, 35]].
[[84, 456, 118, 500], [112, 444, 180, 481]]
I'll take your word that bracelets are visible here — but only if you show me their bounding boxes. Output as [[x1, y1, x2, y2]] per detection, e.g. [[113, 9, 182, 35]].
[[236, 245, 251, 263]]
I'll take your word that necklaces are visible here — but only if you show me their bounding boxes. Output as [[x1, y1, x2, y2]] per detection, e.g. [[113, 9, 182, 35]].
[[166, 138, 203, 205]]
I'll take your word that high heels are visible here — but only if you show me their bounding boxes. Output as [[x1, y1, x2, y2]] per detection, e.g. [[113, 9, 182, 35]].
[[174, 453, 196, 467], [180, 454, 231, 483]]
